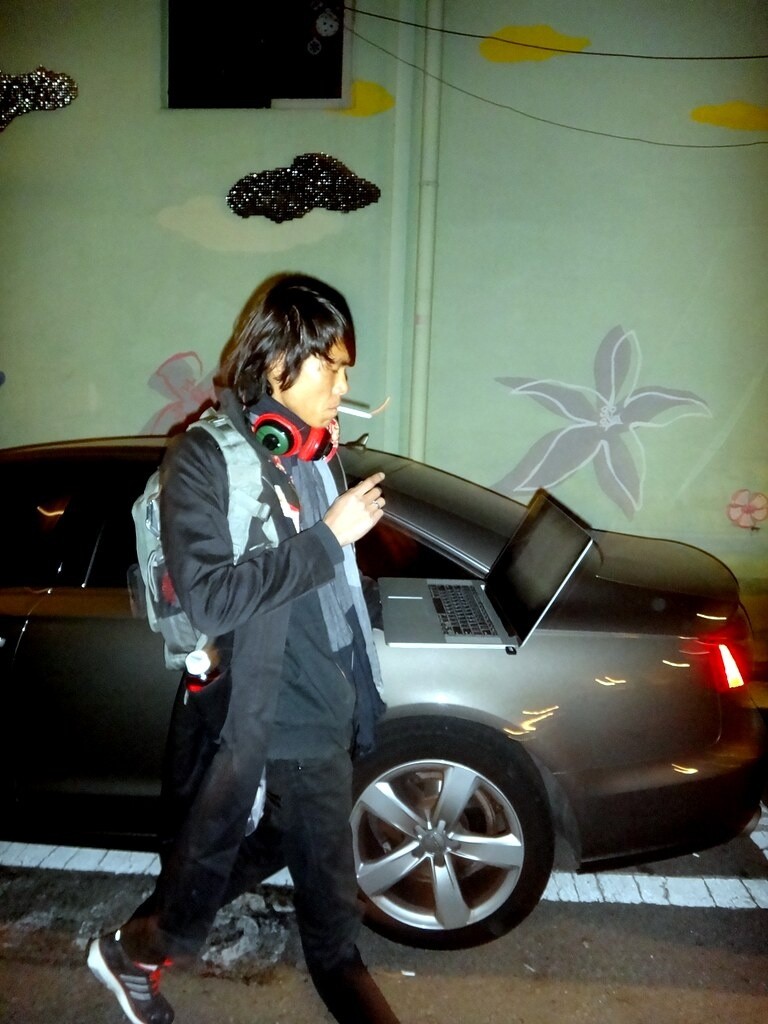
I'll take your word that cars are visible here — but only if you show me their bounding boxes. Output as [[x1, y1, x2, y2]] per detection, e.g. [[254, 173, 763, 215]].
[[0, 434, 767, 953]]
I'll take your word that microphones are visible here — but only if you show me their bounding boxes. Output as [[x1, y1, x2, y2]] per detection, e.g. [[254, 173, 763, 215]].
[[339, 433, 370, 454]]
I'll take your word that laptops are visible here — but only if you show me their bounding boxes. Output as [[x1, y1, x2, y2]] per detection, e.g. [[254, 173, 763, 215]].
[[378, 492, 594, 651]]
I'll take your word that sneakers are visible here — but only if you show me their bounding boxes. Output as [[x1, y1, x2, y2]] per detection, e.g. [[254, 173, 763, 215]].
[[87, 926, 176, 1024]]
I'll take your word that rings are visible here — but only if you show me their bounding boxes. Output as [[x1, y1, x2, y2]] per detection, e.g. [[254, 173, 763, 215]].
[[373, 501, 380, 509]]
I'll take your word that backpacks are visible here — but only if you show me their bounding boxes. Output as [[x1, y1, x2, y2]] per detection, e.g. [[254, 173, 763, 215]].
[[131, 406, 361, 667]]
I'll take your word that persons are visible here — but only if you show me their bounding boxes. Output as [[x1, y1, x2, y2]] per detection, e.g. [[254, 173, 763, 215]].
[[86, 274, 401, 1024]]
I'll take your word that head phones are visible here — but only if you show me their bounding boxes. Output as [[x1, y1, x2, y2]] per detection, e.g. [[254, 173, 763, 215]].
[[244, 410, 370, 462]]
